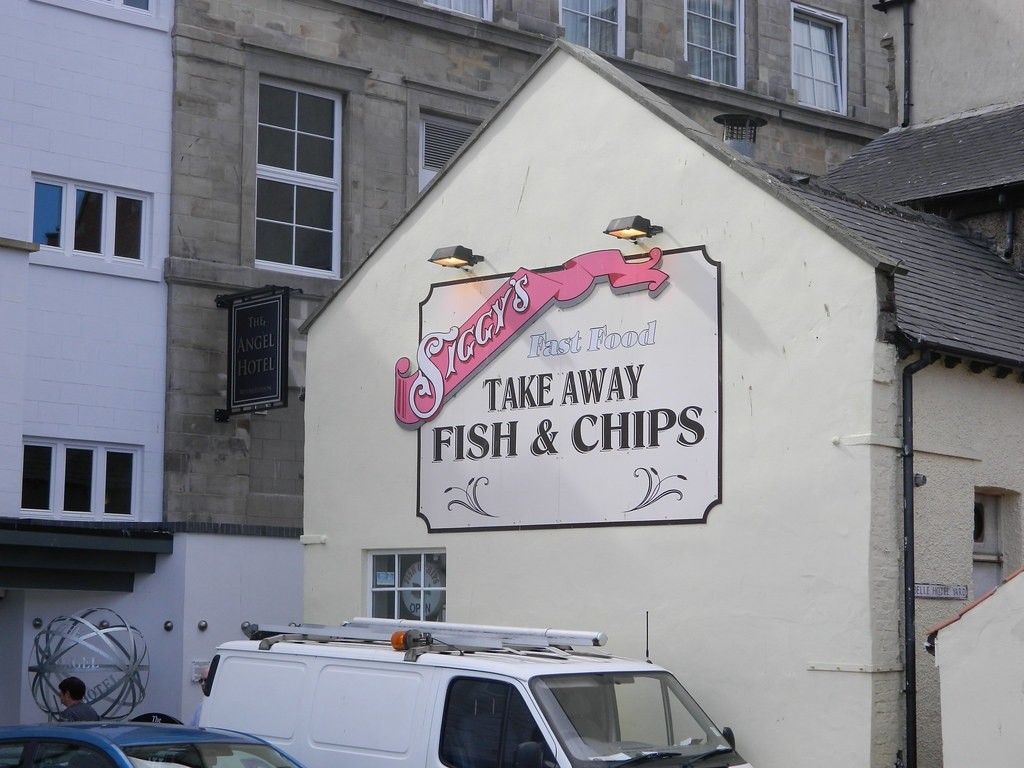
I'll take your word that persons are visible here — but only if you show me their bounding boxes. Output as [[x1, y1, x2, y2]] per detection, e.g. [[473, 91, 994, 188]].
[[57, 677, 100, 722]]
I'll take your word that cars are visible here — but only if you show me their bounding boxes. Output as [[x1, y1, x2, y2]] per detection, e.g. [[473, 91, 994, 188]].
[[3, 722, 301, 768]]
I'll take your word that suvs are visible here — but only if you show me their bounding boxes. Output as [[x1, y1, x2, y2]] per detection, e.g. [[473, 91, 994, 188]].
[[198, 617, 753, 767]]
